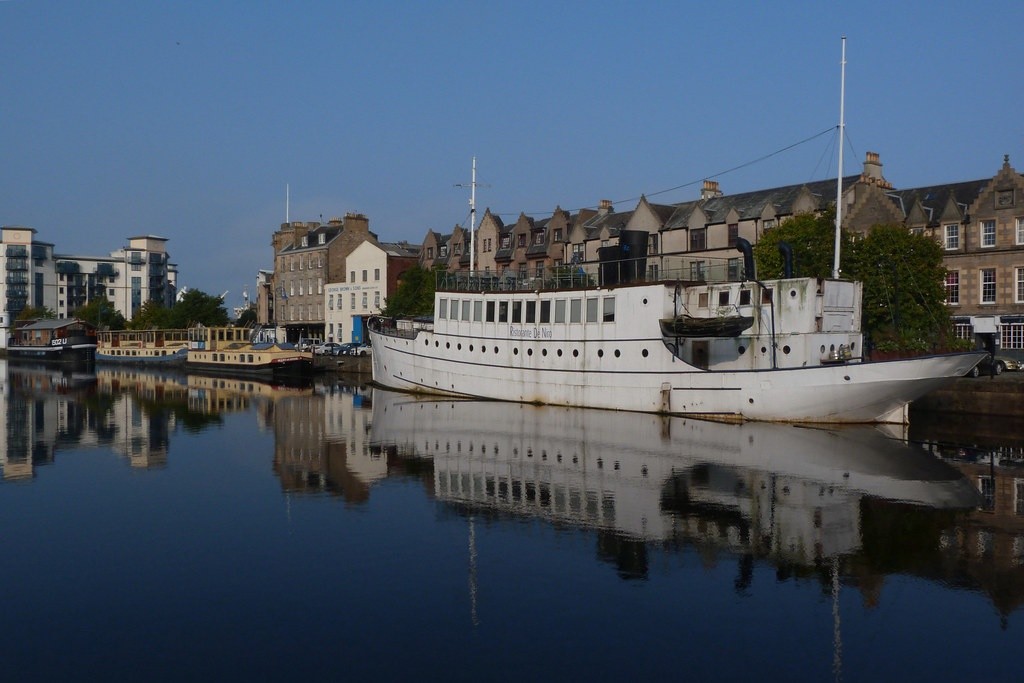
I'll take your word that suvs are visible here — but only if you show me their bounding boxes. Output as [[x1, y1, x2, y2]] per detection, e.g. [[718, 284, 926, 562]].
[[293, 338, 321, 353]]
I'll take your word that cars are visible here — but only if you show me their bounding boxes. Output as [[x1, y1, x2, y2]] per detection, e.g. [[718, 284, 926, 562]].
[[350, 343, 372, 357], [311, 342, 340, 356], [967, 350, 1003, 379], [332, 342, 361, 357], [991, 355, 1023, 373]]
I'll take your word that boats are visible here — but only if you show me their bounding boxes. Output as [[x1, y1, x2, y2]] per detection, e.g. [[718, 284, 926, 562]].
[[95, 328, 188, 369], [186, 364, 314, 399], [6, 319, 98, 362], [366, 35, 992, 428], [186, 326, 315, 374], [96, 365, 189, 397], [366, 387, 989, 683]]
